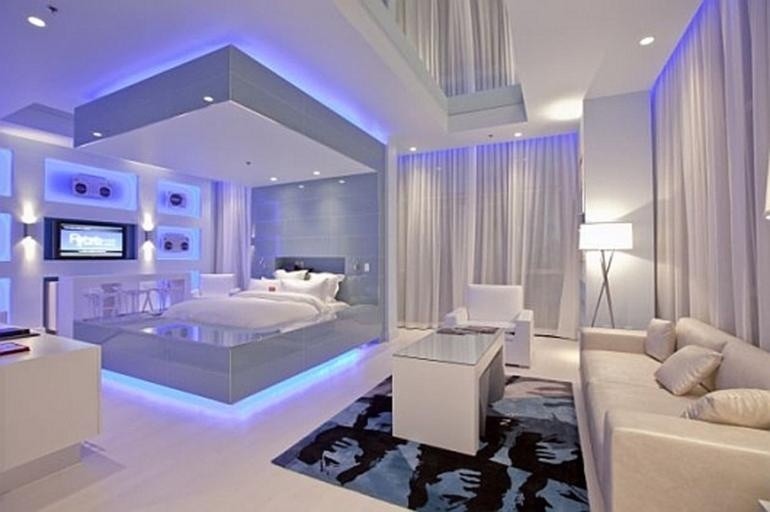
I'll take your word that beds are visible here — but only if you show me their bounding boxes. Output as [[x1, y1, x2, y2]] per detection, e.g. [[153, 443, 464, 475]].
[[72, 257, 383, 404]]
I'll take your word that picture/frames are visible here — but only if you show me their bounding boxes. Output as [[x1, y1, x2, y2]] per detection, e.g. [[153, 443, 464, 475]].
[[43, 158, 139, 211], [0, 148, 14, 198], [0, 278, 11, 324], [156, 226, 201, 261], [0, 213, 12, 263], [157, 179, 201, 220]]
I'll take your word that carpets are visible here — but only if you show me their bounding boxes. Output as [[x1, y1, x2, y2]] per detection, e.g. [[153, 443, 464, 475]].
[[271, 373, 590, 512]]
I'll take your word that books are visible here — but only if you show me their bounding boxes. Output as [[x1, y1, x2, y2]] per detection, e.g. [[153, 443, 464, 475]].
[[455, 324, 500, 334], [436, 326, 470, 336], [0, 341, 31, 356], [0, 332, 41, 340], [0, 326, 30, 336]]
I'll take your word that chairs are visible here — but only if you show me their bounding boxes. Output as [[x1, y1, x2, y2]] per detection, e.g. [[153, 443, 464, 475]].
[[190, 273, 241, 300], [443, 284, 534, 368]]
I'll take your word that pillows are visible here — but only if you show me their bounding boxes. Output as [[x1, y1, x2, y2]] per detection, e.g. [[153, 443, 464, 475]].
[[653, 344, 724, 396], [248, 268, 346, 298], [680, 388, 770, 430], [645, 318, 676, 362]]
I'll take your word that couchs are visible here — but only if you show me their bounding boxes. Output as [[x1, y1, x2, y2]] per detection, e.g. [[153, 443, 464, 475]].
[[579, 317, 770, 512]]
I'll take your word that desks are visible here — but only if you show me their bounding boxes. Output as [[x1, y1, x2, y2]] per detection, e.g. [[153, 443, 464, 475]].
[[0, 324, 102, 474]]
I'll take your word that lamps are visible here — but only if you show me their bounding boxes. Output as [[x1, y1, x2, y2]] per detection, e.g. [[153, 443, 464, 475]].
[[20, 199, 37, 261], [142, 212, 155, 262], [763, 151, 770, 220], [578, 222, 634, 328]]
